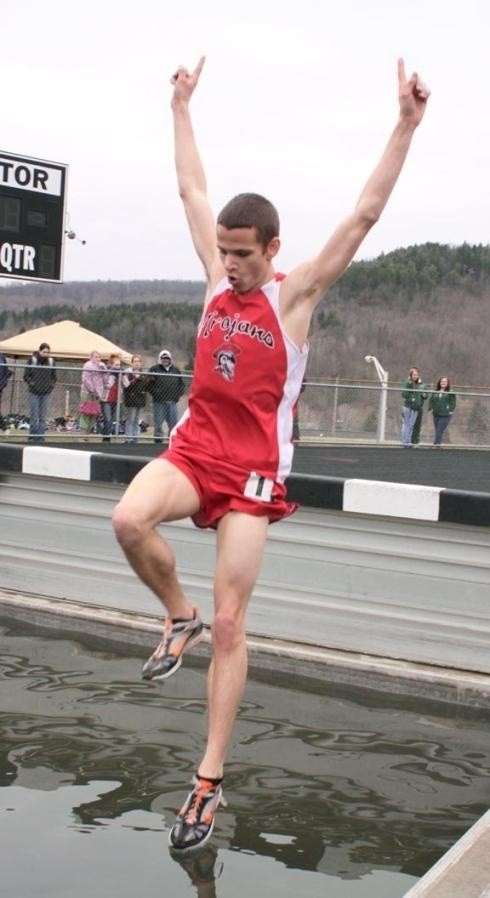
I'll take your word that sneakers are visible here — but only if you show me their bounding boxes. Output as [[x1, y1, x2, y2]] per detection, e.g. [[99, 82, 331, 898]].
[[141, 605, 206, 679], [168, 774, 223, 852]]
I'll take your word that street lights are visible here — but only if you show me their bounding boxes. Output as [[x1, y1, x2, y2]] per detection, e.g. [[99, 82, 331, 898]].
[[363, 354, 389, 442]]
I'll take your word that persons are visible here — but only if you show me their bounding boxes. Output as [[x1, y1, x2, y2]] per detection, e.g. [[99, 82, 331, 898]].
[[291, 377, 309, 440], [402, 368, 455, 449], [115, 56, 449, 849], [1, 342, 186, 443]]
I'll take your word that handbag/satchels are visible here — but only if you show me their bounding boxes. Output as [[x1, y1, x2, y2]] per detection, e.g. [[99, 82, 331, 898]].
[[80, 392, 103, 416]]
